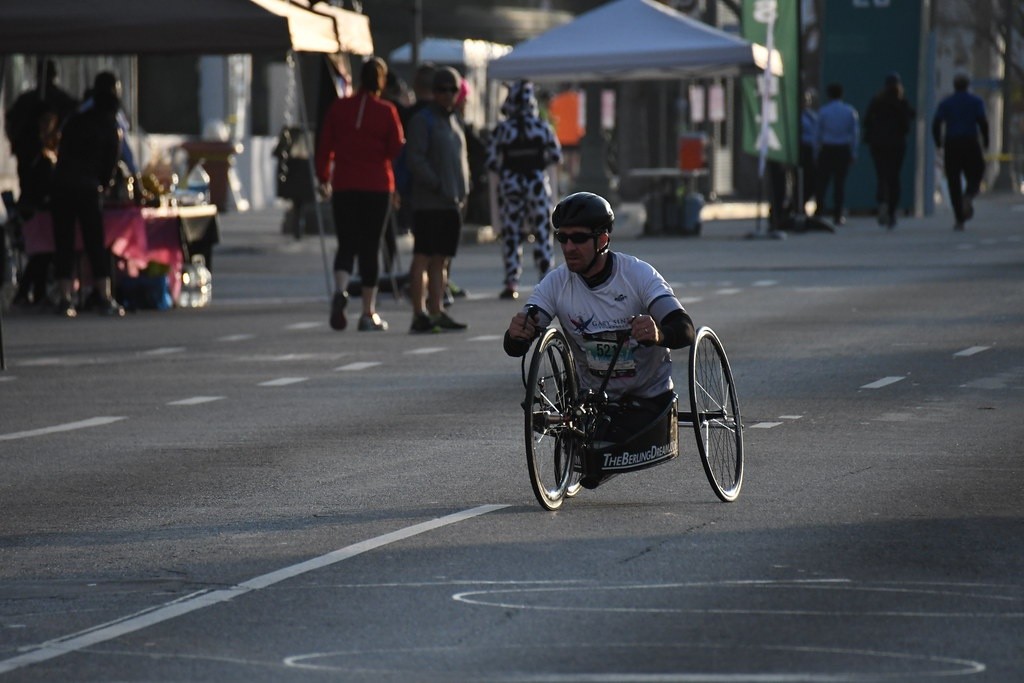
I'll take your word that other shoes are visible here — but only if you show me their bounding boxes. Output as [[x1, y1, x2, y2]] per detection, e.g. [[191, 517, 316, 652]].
[[443, 289, 454, 305], [358, 313, 389, 331], [448, 281, 468, 298], [100, 299, 125, 317], [429, 310, 467, 330], [408, 313, 442, 333], [500, 288, 518, 299], [329, 290, 349, 331]]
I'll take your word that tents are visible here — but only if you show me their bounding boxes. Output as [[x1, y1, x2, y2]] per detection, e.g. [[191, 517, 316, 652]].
[[485, 0, 783, 234], [0, 0, 403, 302]]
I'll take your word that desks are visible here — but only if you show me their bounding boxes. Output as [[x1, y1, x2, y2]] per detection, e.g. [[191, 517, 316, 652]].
[[628, 167, 710, 236], [21, 205, 219, 313]]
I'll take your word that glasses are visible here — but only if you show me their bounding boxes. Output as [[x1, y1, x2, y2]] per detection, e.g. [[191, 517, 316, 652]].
[[438, 85, 458, 93], [554, 231, 604, 244]]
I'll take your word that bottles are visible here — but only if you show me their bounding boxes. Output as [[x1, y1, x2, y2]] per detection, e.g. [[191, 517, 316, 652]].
[[186, 163, 211, 205], [178, 254, 213, 308]]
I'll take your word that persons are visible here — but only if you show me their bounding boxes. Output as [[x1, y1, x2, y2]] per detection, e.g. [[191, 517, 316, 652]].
[[802, 72, 915, 228], [502, 191, 696, 405], [313, 57, 402, 331], [381, 61, 485, 336], [486, 78, 564, 299], [930, 74, 989, 232], [4, 59, 134, 317]]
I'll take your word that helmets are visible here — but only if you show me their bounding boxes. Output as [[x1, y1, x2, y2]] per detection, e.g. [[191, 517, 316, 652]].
[[552, 192, 614, 228]]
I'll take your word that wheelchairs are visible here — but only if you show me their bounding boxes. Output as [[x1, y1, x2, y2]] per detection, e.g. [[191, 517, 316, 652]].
[[511, 305, 746, 511]]
